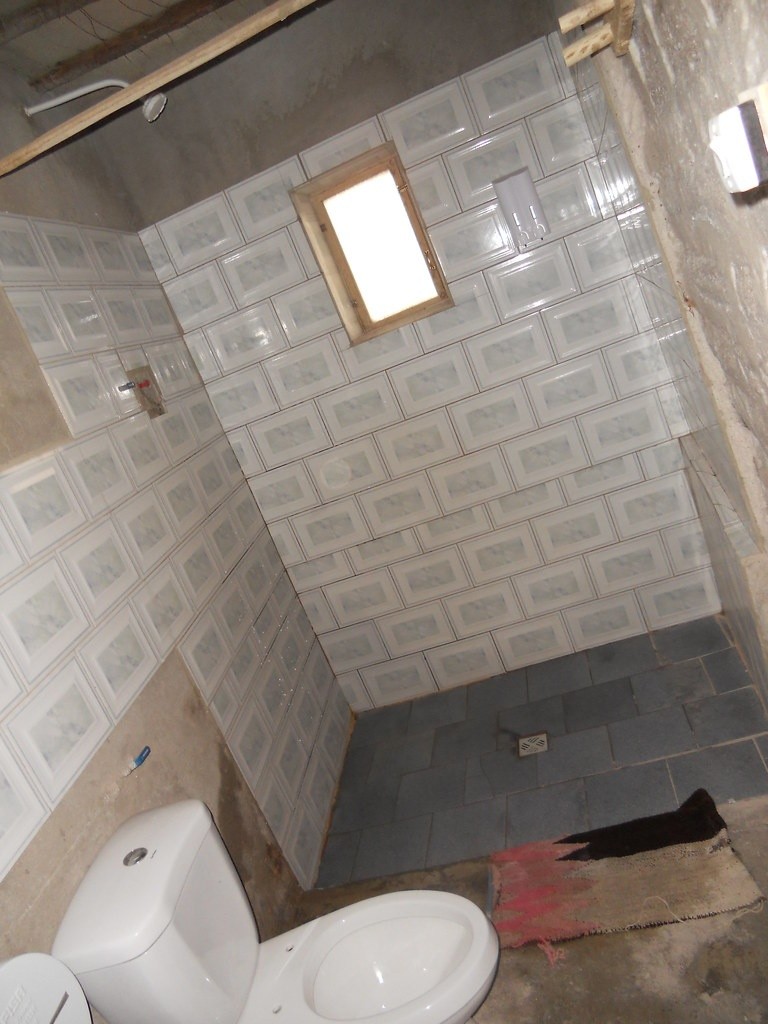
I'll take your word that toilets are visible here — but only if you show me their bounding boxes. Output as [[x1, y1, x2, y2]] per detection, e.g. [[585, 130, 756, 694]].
[[50, 798, 499, 1024]]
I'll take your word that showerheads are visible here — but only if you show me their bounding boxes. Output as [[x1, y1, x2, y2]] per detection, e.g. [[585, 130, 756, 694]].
[[27, 78, 171, 123]]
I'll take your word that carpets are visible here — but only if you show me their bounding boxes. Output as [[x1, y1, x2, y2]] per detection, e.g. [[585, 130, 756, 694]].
[[485, 788, 764, 949]]
